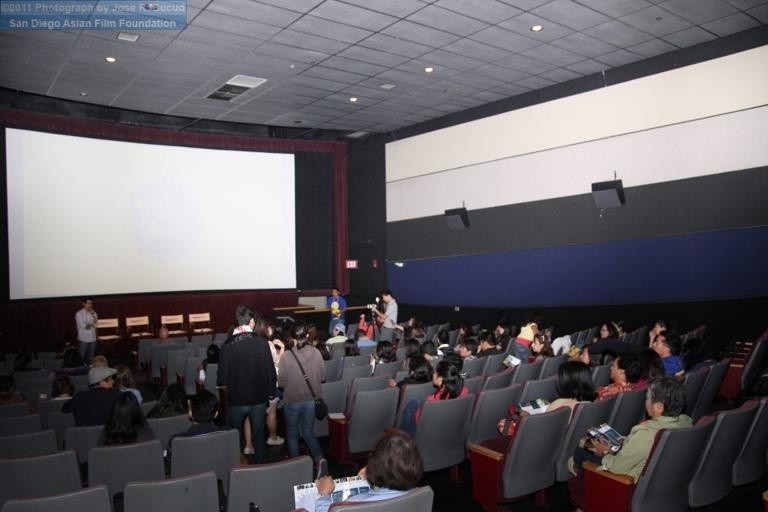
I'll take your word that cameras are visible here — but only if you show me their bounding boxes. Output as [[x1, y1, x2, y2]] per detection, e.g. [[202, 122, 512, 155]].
[[371, 297, 383, 305]]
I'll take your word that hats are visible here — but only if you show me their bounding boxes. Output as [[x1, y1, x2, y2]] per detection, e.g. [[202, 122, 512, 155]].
[[335, 322, 346, 337], [88, 366, 117, 385]]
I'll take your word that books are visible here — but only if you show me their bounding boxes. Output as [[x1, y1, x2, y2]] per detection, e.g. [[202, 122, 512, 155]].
[[583, 422, 623, 452], [517, 397, 551, 416], [293, 475, 371, 512]]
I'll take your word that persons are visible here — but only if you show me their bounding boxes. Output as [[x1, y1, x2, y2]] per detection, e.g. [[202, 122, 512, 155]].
[[116, 364, 142, 404], [74, 299, 99, 364], [367, 339, 396, 378], [97, 391, 159, 449], [398, 319, 437, 359], [315, 431, 427, 512], [145, 383, 188, 419], [571, 380, 694, 482], [566, 317, 628, 367], [357, 311, 378, 347], [372, 288, 397, 344], [437, 317, 554, 389], [594, 348, 660, 404], [196, 343, 219, 401], [165, 389, 223, 479], [278, 322, 329, 479], [47, 348, 90, 383], [325, 323, 353, 359], [387, 357, 433, 413], [241, 316, 285, 454], [62, 367, 120, 428], [48, 374, 74, 400], [396, 360, 469, 439], [327, 287, 347, 338], [505, 362, 595, 450], [89, 356, 106, 368], [218, 304, 285, 465], [650, 321, 722, 381]]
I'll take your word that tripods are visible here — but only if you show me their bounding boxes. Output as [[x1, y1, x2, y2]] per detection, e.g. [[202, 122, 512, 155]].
[[364, 312, 382, 341]]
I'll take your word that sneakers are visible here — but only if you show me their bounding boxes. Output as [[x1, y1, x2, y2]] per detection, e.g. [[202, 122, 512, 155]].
[[244, 447, 255, 455], [267, 436, 285, 445], [315, 457, 329, 480]]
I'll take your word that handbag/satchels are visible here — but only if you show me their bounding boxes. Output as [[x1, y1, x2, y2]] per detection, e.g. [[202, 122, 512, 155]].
[[288, 348, 329, 420]]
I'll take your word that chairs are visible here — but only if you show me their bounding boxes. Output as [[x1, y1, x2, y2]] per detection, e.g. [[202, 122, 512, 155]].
[[570, 326, 597, 346], [482, 365, 516, 389], [556, 398, 618, 481], [683, 326, 706, 346], [229, 455, 313, 511], [328, 387, 399, 464], [682, 374, 706, 411], [698, 358, 731, 416], [347, 374, 393, 418], [1, 401, 30, 413], [423, 323, 461, 346], [0, 431, 59, 461], [520, 377, 557, 404], [374, 360, 404, 377], [89, 439, 165, 496], [140, 399, 158, 417], [0, 414, 41, 432], [461, 375, 482, 407], [330, 486, 434, 512], [397, 380, 439, 431], [137, 332, 227, 398], [731, 329, 768, 376], [541, 354, 570, 377], [583, 416, 716, 511], [608, 389, 647, 438], [45, 413, 73, 443], [731, 398, 767, 487], [149, 414, 189, 453], [2, 485, 112, 511], [322, 357, 340, 382], [36, 400, 64, 430], [624, 325, 647, 347], [512, 359, 544, 383], [410, 394, 474, 482], [593, 364, 617, 388], [0, 449, 82, 499], [170, 428, 241, 498], [467, 408, 571, 510], [480, 352, 510, 377], [461, 357, 485, 377], [124, 473, 219, 511], [66, 426, 106, 467], [312, 380, 348, 438], [3, 350, 89, 398], [686, 400, 758, 509], [469, 383, 524, 448], [338, 356, 371, 380]]
